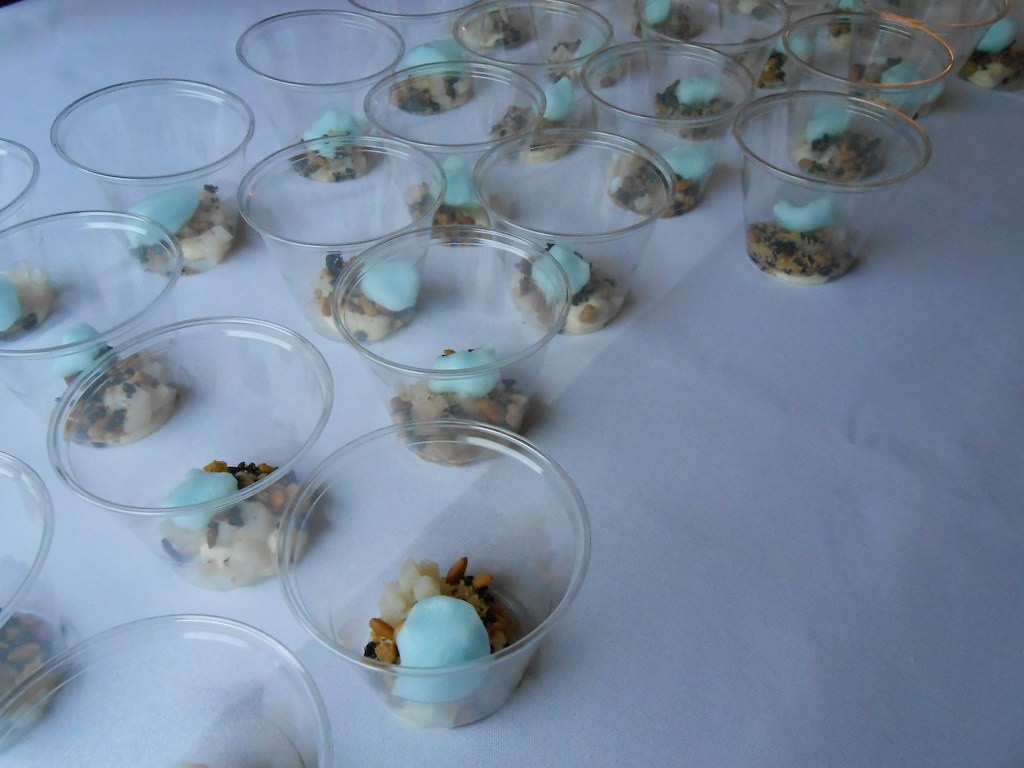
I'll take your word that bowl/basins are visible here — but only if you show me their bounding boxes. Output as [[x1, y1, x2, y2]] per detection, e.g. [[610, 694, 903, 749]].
[[580, 42, 756, 219], [632, 0, 790, 141], [0, 209, 182, 448], [331, 225, 573, 466], [47, 316, 334, 591], [1, 614, 334, 768], [50, 77, 256, 273], [732, 90, 933, 285], [233, 0, 614, 338], [783, 1, 1014, 183], [276, 419, 592, 729], [0, 115, 58, 341], [0, 451, 66, 754], [474, 127, 679, 335]]
